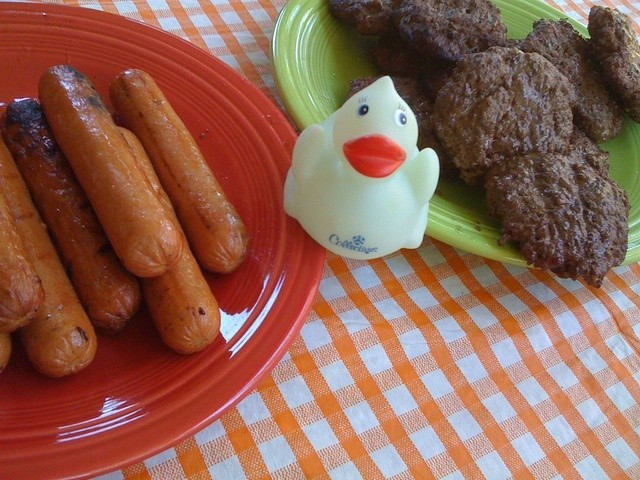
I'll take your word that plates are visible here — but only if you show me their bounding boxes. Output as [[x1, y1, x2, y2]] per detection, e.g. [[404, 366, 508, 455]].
[[0, 1, 325, 476], [270, 0, 640, 269]]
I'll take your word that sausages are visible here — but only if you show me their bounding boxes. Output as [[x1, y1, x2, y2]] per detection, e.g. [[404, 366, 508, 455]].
[[0, 63, 251, 379]]
